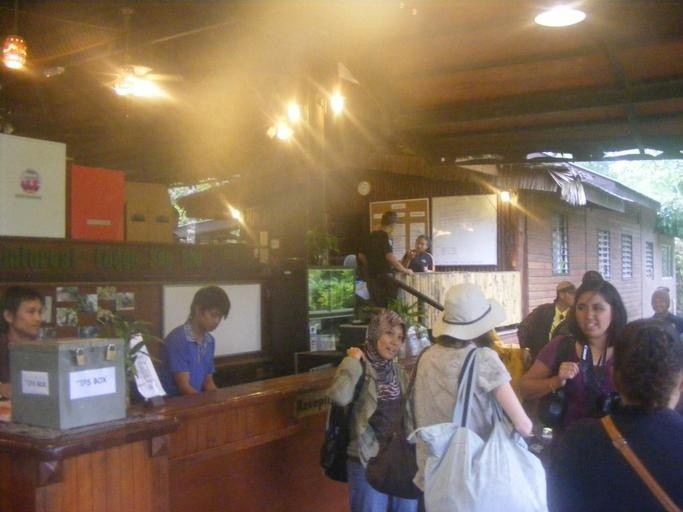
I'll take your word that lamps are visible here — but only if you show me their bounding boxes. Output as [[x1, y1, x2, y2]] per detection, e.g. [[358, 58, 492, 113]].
[[0, 0, 28, 69]]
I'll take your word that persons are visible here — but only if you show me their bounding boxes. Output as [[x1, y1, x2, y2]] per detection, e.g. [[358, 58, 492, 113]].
[[517, 277, 628, 511], [648, 286, 682, 338], [157, 284, 231, 397], [398, 233, 436, 272], [409, 283, 534, 512], [0, 283, 46, 403], [356, 210, 416, 308], [516, 281, 578, 369], [545, 318, 683, 512], [325, 307, 420, 512]]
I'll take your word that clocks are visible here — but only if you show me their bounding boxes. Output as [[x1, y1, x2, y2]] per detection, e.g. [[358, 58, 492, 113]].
[[355, 179, 372, 198]]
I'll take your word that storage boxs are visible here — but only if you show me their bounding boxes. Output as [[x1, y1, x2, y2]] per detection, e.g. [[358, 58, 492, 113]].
[[124, 180, 172, 243], [0, 133, 67, 239], [67, 161, 125, 242]]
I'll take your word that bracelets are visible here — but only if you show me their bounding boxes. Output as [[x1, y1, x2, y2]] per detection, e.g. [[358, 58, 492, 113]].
[[520, 345, 530, 351], [547, 378, 555, 394]]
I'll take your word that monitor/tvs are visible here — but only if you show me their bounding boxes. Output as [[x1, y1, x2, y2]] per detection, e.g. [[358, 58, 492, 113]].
[[291, 351, 342, 376]]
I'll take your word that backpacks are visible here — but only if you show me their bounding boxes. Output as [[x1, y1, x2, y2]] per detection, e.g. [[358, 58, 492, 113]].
[[319, 349, 365, 486]]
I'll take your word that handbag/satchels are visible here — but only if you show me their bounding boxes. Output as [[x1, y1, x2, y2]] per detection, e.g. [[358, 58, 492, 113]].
[[405, 417, 551, 511], [535, 331, 575, 430], [364, 427, 422, 500]]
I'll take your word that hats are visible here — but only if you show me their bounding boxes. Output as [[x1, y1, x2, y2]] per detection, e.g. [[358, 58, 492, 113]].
[[430, 281, 508, 341], [556, 280, 575, 292], [650, 286, 670, 305]]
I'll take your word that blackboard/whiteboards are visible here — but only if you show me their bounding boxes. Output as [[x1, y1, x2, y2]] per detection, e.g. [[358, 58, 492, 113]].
[[430, 194, 498, 267], [162, 284, 261, 358]]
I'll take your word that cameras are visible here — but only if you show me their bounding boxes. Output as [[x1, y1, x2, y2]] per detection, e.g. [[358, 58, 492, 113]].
[[601, 389, 622, 416]]
